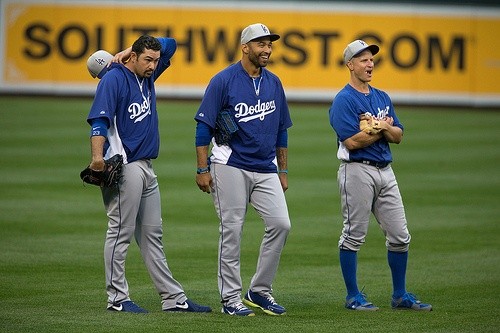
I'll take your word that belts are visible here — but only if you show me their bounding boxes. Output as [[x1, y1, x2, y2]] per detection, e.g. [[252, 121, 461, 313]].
[[341, 159, 389, 169]]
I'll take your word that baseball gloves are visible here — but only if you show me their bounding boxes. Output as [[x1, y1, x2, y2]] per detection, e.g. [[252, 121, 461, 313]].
[[214, 111, 239, 146], [80, 153, 123, 187]]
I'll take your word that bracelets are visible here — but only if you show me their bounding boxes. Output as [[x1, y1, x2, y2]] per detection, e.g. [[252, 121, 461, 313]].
[[279, 169, 289, 174], [196, 167, 210, 175]]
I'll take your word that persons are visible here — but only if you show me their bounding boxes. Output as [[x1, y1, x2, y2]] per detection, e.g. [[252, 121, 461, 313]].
[[328, 39, 435, 311], [80, 35, 213, 314], [194, 23, 292, 317]]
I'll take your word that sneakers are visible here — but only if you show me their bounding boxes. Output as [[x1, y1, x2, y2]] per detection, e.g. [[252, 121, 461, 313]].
[[163, 299, 212, 313], [222, 302, 255, 316], [107, 301, 148, 313], [391, 292, 432, 312], [242, 288, 286, 316], [345, 286, 380, 311]]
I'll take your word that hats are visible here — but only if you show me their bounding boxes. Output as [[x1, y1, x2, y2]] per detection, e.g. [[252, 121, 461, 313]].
[[344, 40, 379, 63], [241, 23, 280, 45], [87, 50, 115, 78]]
